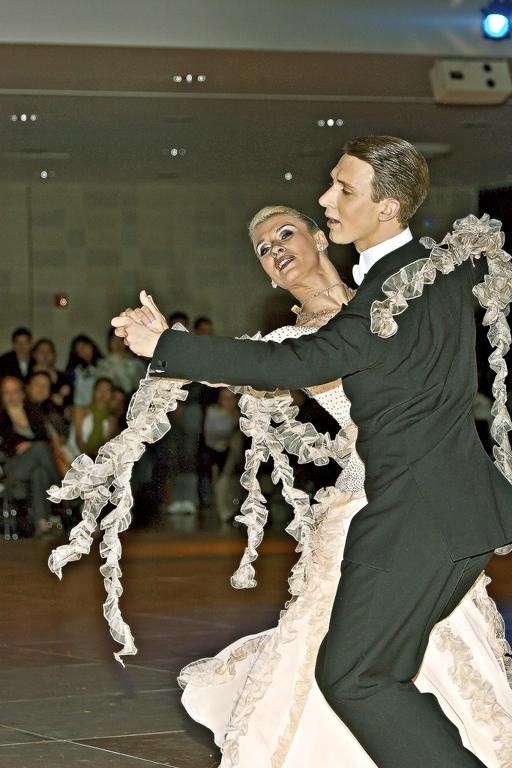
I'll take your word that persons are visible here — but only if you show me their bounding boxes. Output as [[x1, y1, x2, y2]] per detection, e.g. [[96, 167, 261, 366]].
[[104, 128, 512, 766], [0, 312, 343, 538], [41, 203, 512, 766]]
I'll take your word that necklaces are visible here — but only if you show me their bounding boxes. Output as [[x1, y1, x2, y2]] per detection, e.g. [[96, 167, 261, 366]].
[[289, 311, 333, 323], [296, 280, 351, 310]]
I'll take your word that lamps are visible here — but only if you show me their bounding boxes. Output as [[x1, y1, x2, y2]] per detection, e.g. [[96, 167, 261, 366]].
[[478, 0, 509, 44]]
